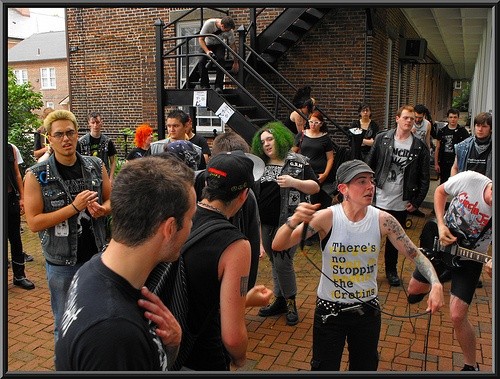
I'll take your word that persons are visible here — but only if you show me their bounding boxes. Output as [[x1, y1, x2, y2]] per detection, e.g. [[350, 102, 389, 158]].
[[409, 170, 492, 372], [54, 157, 197, 372], [271, 160, 444, 372], [194, 133, 261, 293], [145, 151, 273, 372], [162, 140, 202, 171], [76, 112, 117, 177], [283, 97, 334, 182], [6, 142, 34, 290], [251, 121, 321, 325], [349, 103, 492, 286], [22, 110, 112, 344], [34, 108, 54, 162], [199, 16, 239, 90], [166, 110, 211, 165], [125, 125, 153, 160]]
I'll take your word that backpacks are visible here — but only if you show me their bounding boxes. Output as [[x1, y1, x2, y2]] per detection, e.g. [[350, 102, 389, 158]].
[[143, 219, 239, 332]]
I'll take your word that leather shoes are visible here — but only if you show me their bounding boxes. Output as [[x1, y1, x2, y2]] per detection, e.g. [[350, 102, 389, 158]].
[[258, 296, 287, 317], [13, 277, 35, 290], [286, 298, 299, 325]]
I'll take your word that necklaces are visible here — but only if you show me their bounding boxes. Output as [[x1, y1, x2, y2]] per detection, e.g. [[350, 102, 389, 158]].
[[198, 200, 228, 219]]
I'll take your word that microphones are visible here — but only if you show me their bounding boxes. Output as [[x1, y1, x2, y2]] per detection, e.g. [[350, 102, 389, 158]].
[[301, 222, 309, 250]]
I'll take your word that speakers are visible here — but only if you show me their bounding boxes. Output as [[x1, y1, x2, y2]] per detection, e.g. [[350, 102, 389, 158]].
[[403, 38, 427, 59]]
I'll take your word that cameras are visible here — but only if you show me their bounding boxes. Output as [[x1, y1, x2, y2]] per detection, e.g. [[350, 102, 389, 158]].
[[205, 53, 219, 69]]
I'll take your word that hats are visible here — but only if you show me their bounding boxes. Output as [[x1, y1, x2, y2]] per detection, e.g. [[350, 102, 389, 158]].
[[204, 149, 265, 193], [336, 160, 375, 184]]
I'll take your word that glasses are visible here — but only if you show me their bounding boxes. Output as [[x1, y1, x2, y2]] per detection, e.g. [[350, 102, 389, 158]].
[[51, 130, 76, 139], [308, 120, 321, 125]]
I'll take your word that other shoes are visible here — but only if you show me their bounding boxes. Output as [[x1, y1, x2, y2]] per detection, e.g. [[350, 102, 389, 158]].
[[306, 239, 313, 246], [430, 209, 436, 216], [409, 209, 425, 218], [476, 279, 483, 288], [386, 274, 400, 286], [460, 362, 480, 372], [408, 291, 430, 303], [22, 252, 33, 262]]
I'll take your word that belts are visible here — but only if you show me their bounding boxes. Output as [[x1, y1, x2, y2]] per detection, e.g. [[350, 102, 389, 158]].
[[317, 298, 380, 316]]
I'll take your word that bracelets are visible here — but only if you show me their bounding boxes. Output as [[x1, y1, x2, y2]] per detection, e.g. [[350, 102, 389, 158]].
[[72, 203, 80, 212], [286, 216, 295, 230]]
